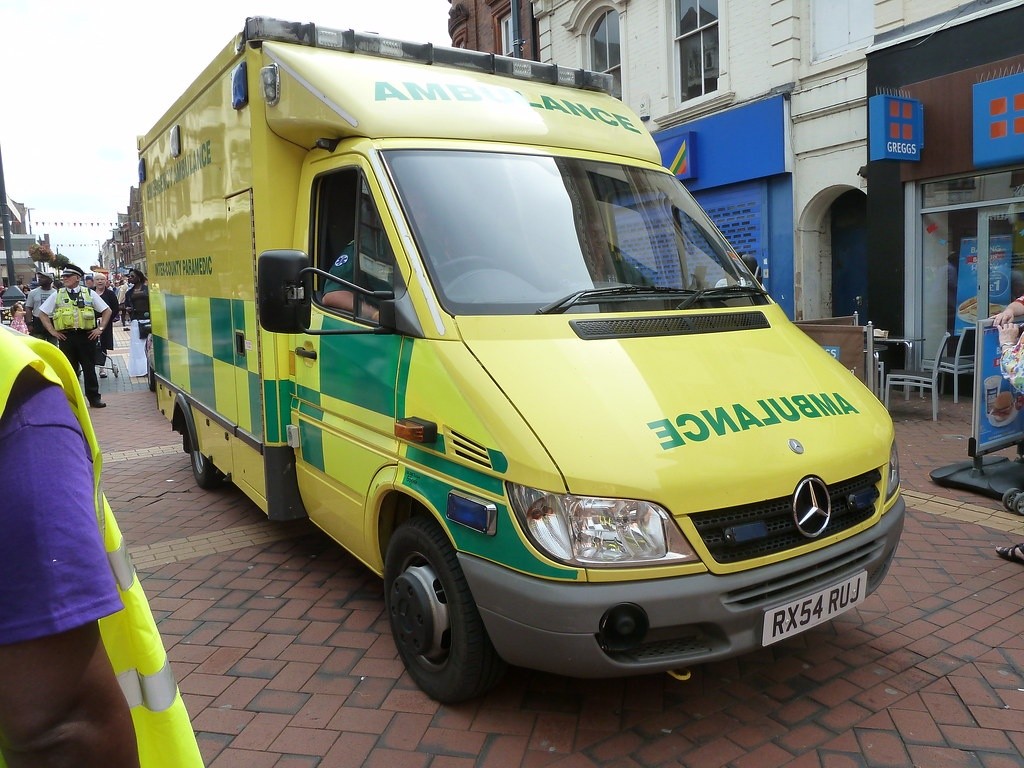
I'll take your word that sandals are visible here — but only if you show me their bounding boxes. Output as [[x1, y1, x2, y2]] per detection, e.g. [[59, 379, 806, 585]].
[[994, 542, 1024, 565]]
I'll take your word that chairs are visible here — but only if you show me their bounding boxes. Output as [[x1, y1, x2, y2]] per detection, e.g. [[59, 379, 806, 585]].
[[884, 331, 951, 420], [919, 326, 976, 404]]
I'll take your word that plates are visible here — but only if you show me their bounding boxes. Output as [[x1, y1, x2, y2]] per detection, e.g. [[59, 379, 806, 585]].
[[958, 304, 1006, 324], [988, 407, 1018, 426]]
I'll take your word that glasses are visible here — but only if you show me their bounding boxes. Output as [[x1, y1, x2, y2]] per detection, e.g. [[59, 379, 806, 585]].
[[127, 274, 138, 278]]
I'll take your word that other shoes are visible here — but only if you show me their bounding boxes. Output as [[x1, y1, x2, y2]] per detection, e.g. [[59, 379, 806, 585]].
[[99, 369, 107, 378]]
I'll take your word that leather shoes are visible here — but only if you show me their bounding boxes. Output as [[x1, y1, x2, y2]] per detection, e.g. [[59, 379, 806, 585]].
[[90, 400, 106, 408]]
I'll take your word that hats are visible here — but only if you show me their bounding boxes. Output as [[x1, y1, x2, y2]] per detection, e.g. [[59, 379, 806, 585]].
[[36, 271, 55, 282], [60, 264, 85, 277]]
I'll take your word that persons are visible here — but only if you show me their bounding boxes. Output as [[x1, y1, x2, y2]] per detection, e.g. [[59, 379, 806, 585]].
[[573, 205, 657, 288], [0, 323, 205, 768], [26, 271, 57, 347], [33, 263, 112, 408], [0, 283, 31, 298], [322, 225, 401, 323], [988, 294, 1024, 568], [125, 268, 152, 378], [90, 272, 118, 378], [75, 276, 134, 331], [10, 304, 27, 334]]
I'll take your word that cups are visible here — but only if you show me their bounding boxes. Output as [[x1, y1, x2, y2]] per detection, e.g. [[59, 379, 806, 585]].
[[984, 375, 1002, 417]]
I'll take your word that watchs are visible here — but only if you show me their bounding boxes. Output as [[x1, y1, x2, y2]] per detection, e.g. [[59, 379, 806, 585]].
[[98, 326, 104, 331]]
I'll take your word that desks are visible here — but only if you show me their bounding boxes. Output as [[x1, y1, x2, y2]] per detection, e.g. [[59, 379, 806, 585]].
[[864, 333, 927, 401]]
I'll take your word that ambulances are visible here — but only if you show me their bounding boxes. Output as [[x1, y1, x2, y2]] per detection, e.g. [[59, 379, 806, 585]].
[[137, 17, 907, 704]]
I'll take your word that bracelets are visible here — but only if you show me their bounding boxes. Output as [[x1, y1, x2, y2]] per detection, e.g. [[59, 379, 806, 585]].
[[372, 310, 380, 321]]
